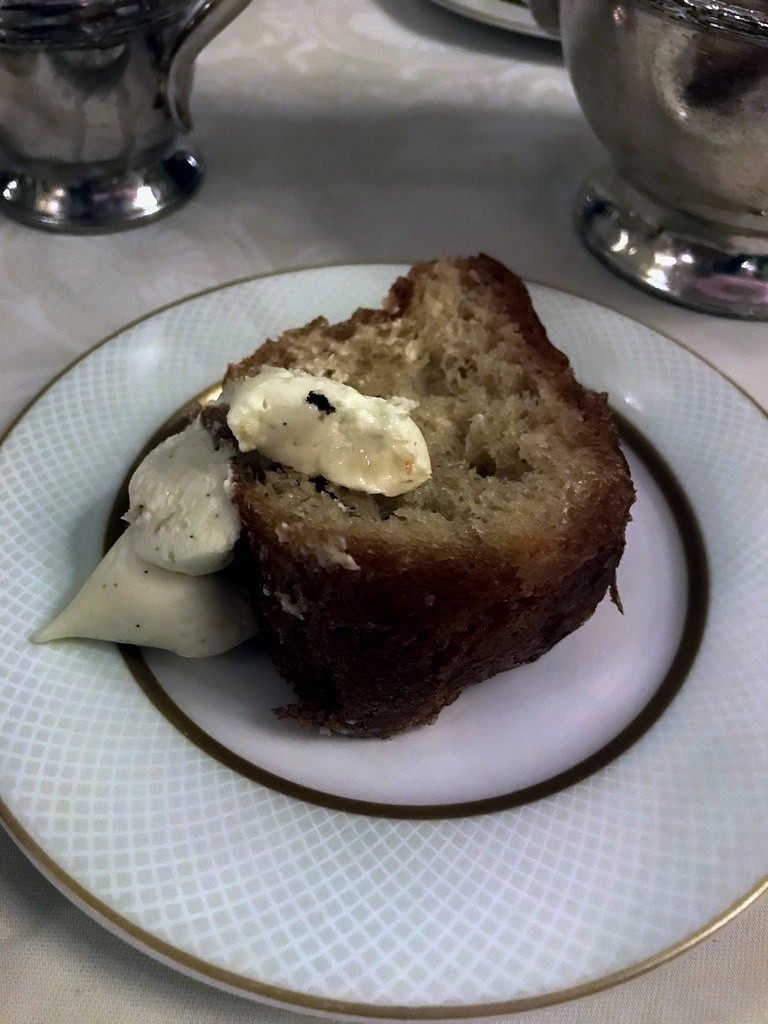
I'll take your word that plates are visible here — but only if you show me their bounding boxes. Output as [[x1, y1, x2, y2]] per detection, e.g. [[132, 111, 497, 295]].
[[0, 263, 768, 1023], [432, 0, 558, 41]]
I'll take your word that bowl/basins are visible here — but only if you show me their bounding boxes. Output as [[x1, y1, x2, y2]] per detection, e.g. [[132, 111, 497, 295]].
[[556, 0, 768, 319], [0, 0, 252, 234]]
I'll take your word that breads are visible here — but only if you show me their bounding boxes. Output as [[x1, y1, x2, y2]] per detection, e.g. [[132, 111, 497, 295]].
[[221, 252, 635, 736]]
